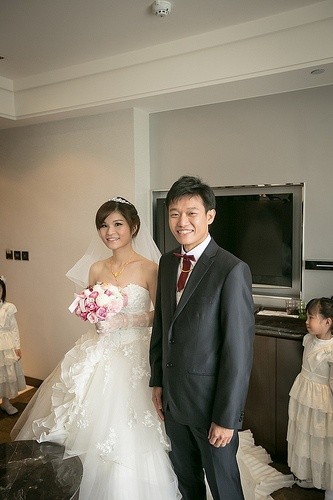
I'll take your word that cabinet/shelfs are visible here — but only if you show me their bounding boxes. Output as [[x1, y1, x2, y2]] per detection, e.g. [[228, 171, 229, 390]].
[[241, 328, 303, 465]]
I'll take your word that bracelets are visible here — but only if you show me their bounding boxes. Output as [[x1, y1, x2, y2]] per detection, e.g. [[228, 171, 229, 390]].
[[121, 312, 129, 331]]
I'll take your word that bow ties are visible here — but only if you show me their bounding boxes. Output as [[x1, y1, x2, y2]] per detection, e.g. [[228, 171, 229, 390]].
[[173, 251, 196, 263]]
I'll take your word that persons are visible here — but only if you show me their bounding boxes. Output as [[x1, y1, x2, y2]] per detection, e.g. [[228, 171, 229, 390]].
[[0, 275, 26, 415], [286, 297, 333, 500], [55, 196, 181, 500], [149, 176, 255, 500]]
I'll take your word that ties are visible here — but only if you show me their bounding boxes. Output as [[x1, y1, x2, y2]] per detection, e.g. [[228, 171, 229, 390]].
[[177, 259, 191, 293]]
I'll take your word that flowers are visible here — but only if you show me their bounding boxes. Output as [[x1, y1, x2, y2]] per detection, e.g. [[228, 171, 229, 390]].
[[68, 281, 129, 325]]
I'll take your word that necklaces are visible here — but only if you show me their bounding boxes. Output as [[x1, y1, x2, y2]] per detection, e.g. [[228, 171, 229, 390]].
[[180, 258, 194, 273], [111, 253, 138, 281]]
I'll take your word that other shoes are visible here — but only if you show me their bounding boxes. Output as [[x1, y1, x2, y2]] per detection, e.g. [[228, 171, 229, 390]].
[[296, 480, 316, 488], [0, 402, 19, 415]]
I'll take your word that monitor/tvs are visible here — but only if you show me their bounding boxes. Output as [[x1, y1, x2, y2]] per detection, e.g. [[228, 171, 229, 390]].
[[152, 184, 303, 309]]
